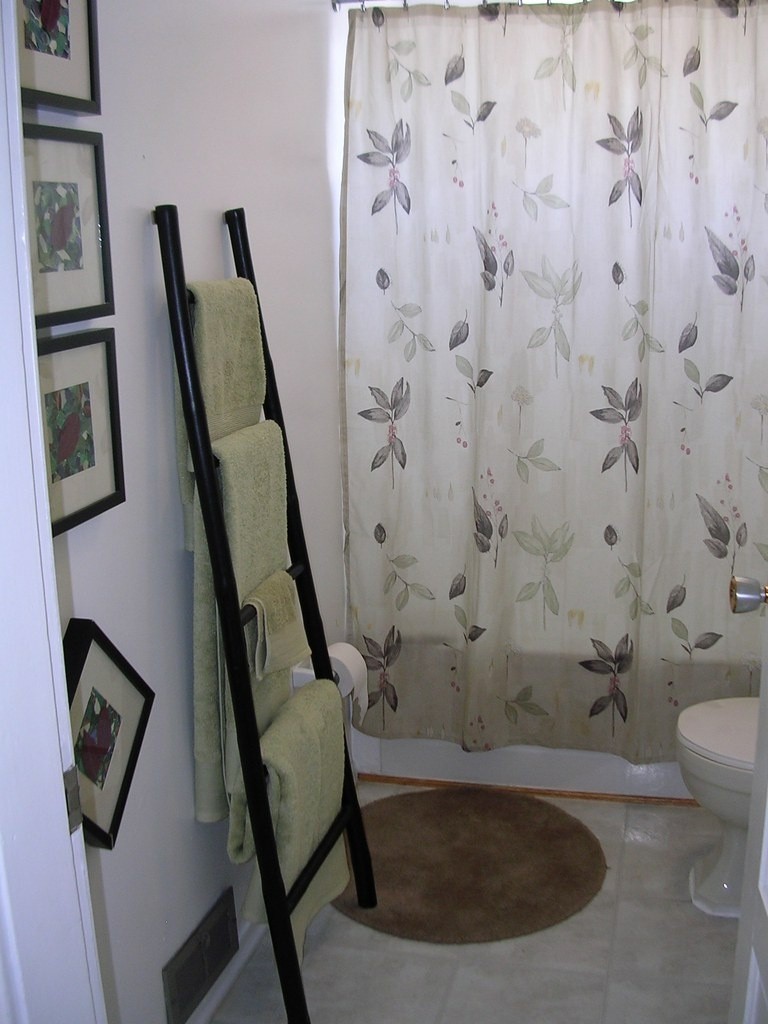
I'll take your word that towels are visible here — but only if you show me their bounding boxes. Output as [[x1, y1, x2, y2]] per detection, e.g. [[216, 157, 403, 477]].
[[191, 419, 289, 824], [226, 677, 352, 972], [172, 277, 267, 552], [224, 568, 314, 795]]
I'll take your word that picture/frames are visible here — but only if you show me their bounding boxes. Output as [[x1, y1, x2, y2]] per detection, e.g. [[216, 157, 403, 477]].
[[63, 617, 156, 851], [37, 327, 126, 538], [16, 0, 102, 118], [22, 122, 116, 330]]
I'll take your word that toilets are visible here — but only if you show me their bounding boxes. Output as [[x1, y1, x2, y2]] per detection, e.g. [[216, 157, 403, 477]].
[[673, 696, 759, 919]]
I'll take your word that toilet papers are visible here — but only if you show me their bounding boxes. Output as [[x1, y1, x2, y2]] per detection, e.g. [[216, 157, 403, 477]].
[[327, 642, 369, 726]]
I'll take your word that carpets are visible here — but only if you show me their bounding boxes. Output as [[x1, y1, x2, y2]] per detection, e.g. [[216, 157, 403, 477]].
[[330, 785, 608, 944]]
